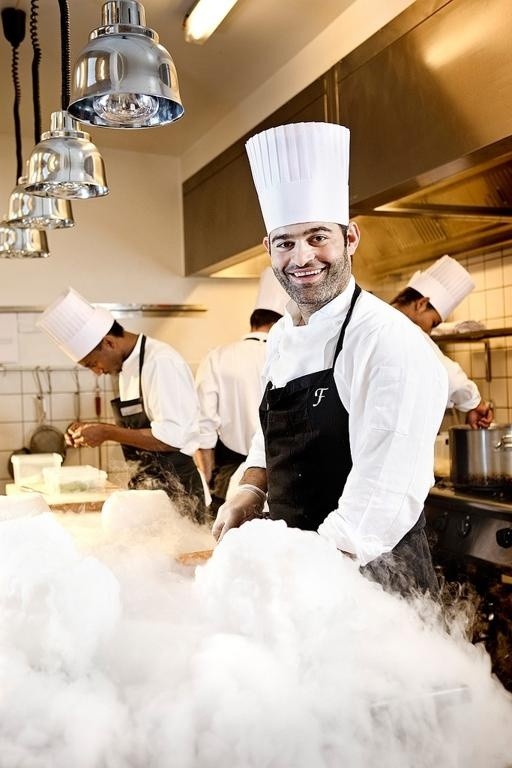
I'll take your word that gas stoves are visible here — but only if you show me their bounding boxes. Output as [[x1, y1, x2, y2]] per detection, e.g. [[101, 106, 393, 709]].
[[415, 479, 512, 567]]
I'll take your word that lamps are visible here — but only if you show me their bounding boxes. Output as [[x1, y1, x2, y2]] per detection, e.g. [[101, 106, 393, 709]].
[[1, 1, 77, 230], [1, 8, 52, 259], [67, 1, 184, 128], [182, 0, 239, 46], [24, 1, 110, 200]]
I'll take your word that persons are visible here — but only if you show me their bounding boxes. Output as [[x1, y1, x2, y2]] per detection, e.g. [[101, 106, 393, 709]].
[[197, 308, 285, 517], [63, 319, 214, 524], [214, 220, 446, 635], [388, 287, 494, 430]]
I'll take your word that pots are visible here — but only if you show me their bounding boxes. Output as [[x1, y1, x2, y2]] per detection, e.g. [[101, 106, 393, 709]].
[[29, 395, 67, 465], [448, 423, 512, 494]]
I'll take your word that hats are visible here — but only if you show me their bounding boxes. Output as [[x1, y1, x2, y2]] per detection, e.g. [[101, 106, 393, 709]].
[[244, 122, 351, 238], [408, 253, 477, 322], [36, 285, 116, 365], [253, 266, 292, 318]]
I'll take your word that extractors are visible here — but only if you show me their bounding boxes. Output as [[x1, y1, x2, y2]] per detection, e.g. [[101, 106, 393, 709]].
[[181, 2, 512, 282]]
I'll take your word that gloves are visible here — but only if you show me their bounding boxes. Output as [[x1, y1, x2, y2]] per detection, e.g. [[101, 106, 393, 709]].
[[211, 483, 268, 544]]
